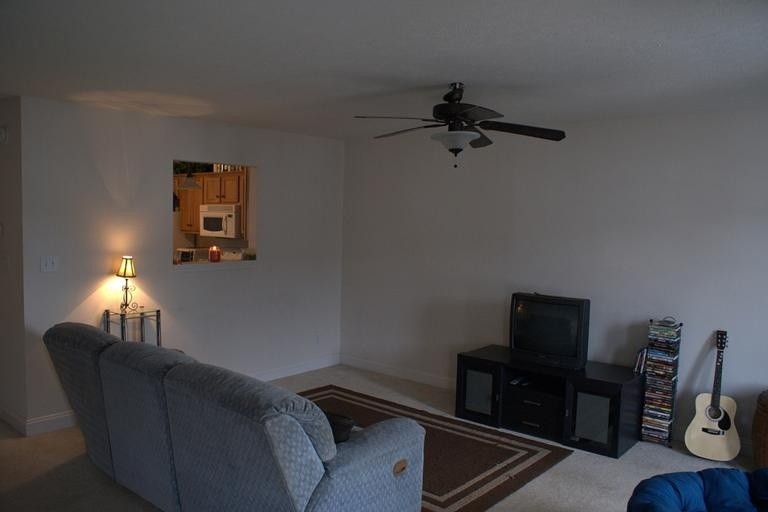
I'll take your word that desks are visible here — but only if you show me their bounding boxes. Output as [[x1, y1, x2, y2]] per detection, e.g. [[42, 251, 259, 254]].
[[102, 306, 162, 350]]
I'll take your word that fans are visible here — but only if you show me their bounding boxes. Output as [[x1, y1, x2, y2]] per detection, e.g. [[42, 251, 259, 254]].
[[337, 81, 566, 148]]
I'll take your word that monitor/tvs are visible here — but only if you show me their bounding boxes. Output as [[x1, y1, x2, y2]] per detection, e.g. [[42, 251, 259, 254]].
[[509, 292, 590, 371]]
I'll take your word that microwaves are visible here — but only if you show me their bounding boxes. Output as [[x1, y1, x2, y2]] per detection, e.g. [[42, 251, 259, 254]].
[[199, 205, 241, 240]]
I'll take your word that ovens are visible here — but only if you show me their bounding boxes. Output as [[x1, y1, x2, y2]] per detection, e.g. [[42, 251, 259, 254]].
[[174, 248, 208, 264]]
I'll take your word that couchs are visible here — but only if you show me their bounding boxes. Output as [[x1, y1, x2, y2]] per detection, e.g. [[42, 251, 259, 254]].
[[42, 320, 427, 512]]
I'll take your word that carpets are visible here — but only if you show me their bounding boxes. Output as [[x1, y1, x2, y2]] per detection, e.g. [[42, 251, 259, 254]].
[[290, 384, 575, 512]]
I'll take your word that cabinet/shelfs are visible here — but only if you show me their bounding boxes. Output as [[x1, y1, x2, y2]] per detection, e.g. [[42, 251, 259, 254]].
[[203, 173, 239, 204], [454, 342, 644, 461], [175, 173, 201, 234]]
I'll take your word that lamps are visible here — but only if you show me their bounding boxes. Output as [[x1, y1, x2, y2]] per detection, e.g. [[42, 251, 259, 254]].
[[430, 85, 481, 157], [117, 255, 139, 311]]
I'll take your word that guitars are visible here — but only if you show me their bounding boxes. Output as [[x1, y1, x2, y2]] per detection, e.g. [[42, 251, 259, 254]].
[[684, 331, 741, 462]]
